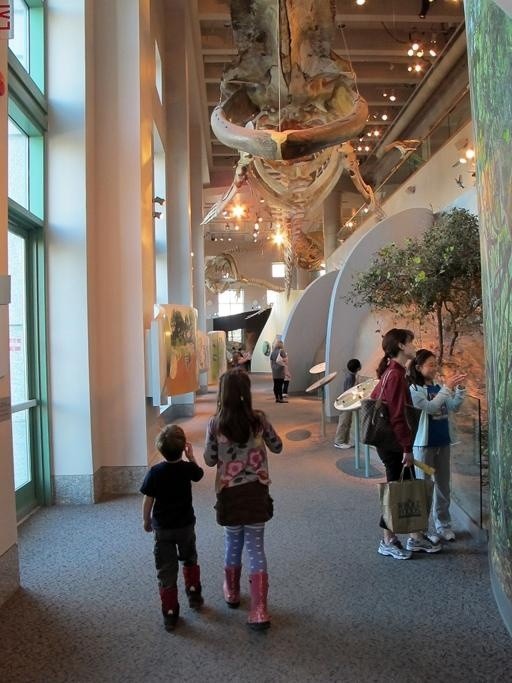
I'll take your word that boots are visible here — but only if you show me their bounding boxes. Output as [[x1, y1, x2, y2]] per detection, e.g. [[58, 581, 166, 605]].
[[247, 573, 272, 631], [183, 564, 205, 609], [157, 584, 180, 633], [274, 393, 289, 403], [222, 562, 239, 604]]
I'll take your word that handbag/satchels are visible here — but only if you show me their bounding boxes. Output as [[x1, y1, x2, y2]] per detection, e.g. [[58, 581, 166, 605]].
[[276, 353, 288, 366], [377, 480, 432, 534], [360, 399, 421, 452]]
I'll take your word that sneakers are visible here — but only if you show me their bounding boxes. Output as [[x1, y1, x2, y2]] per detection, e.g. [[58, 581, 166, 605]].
[[377, 528, 455, 559], [334, 441, 354, 449]]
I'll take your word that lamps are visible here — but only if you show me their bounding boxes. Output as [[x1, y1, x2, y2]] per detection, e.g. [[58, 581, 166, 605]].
[[153, 192, 170, 221], [403, 34, 443, 61]]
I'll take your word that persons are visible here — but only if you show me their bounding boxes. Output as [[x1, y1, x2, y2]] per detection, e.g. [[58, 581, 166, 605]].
[[369, 326, 444, 561], [201, 368, 286, 633], [407, 348, 470, 547], [281, 359, 292, 398], [136, 423, 204, 633], [270, 339, 288, 403], [331, 358, 362, 450]]
